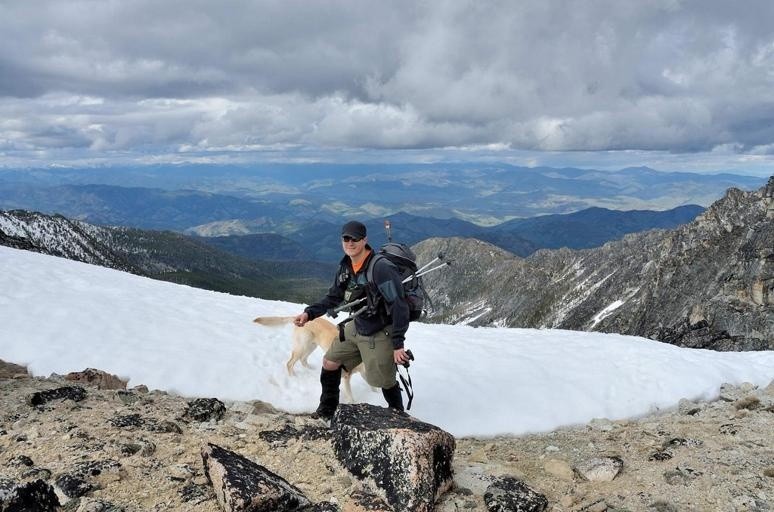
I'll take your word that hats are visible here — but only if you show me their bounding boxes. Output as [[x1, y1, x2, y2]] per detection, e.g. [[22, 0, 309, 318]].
[[342, 221, 366, 240]]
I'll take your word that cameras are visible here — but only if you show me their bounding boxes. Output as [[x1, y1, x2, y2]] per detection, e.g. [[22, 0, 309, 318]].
[[402, 350, 414, 368]]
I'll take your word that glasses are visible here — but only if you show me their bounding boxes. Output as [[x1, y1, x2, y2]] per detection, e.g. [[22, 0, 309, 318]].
[[343, 236, 363, 242]]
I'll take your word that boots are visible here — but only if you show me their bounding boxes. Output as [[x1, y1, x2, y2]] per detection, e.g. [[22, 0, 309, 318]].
[[311, 367, 341, 419]]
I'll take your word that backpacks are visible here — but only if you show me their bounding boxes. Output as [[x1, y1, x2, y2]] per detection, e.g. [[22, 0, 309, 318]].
[[366, 243, 423, 322]]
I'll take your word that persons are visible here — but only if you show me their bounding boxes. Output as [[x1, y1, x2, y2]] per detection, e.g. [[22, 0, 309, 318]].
[[290, 220, 410, 421]]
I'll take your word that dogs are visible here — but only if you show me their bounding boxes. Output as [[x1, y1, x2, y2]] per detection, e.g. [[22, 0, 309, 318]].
[[253, 315, 377, 405]]
